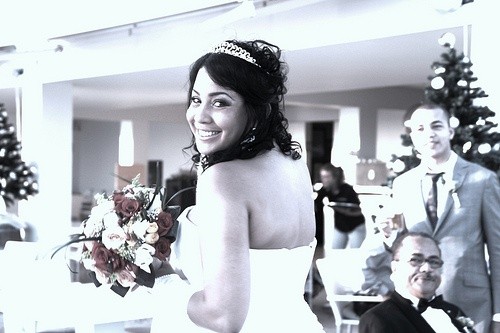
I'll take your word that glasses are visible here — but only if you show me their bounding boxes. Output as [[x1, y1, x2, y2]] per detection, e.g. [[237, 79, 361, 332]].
[[410, 256, 444, 268]]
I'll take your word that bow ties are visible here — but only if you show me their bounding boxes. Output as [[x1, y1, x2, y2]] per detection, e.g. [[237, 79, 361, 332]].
[[417, 294, 443, 314]]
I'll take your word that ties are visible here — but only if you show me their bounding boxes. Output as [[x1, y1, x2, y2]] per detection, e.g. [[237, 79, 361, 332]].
[[425, 172, 446, 226]]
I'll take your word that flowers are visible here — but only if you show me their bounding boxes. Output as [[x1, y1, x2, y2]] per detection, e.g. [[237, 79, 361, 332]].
[[51, 160, 197, 297]]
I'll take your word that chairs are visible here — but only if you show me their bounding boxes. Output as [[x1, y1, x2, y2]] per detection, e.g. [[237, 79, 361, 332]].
[[316, 259, 390, 333]]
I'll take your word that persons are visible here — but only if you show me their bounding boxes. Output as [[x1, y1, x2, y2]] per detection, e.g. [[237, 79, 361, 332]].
[[358, 231, 477, 333], [315, 163, 366, 249], [393, 103, 500, 333], [150, 37, 323, 333]]
